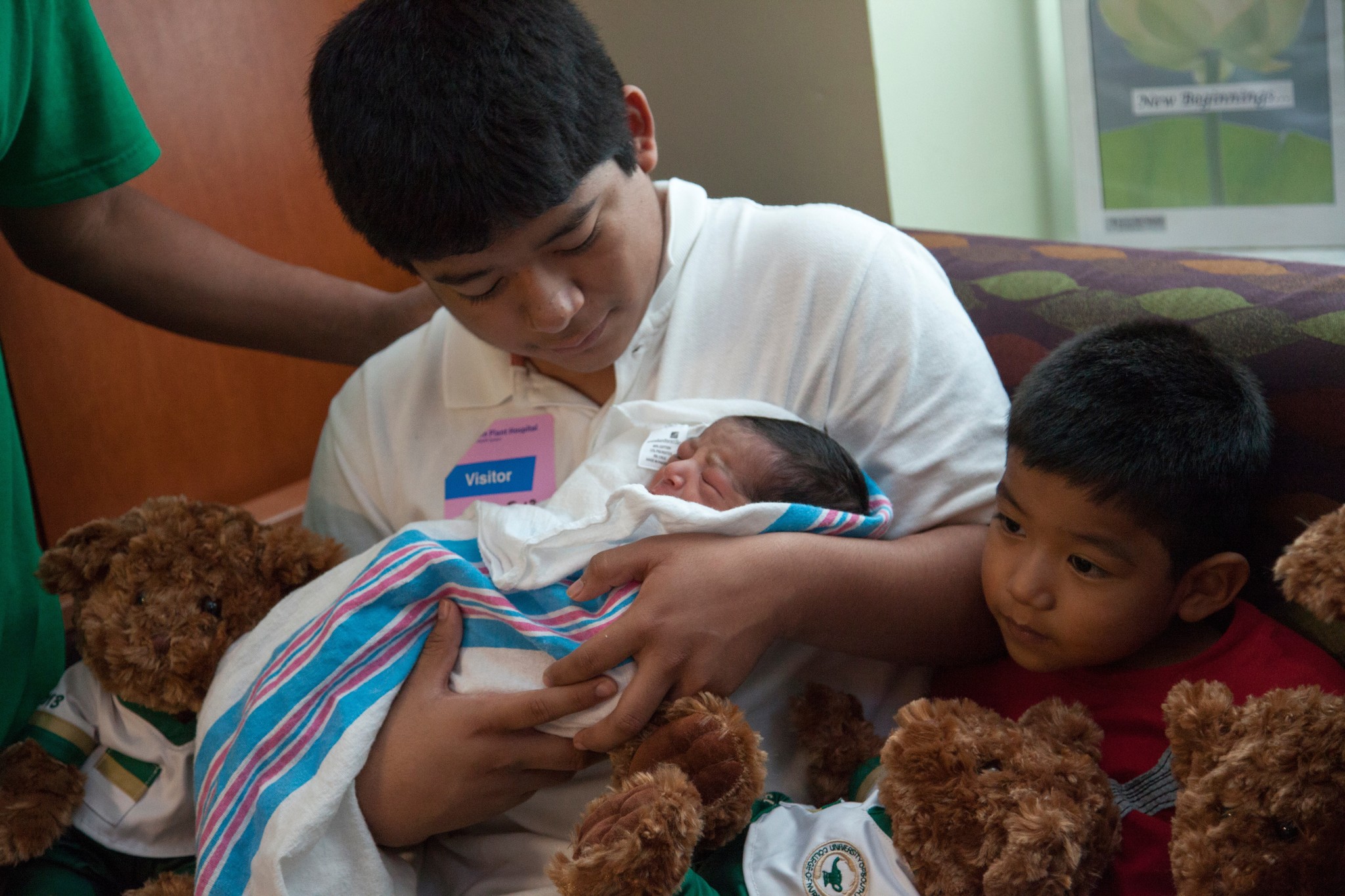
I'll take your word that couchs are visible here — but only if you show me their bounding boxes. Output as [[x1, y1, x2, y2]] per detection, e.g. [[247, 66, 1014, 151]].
[[896, 224, 1345, 627]]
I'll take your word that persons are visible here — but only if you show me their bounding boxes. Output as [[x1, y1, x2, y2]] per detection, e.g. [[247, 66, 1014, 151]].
[[0, 0, 449, 766], [932, 312, 1344, 896], [298, 0, 1016, 896], [364, 414, 878, 742]]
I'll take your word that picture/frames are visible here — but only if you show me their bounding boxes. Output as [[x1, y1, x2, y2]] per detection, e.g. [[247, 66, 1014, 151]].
[[1058, 0, 1345, 258]]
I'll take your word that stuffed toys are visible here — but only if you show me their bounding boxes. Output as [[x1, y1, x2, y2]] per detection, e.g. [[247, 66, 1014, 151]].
[[0, 489, 351, 896], [1271, 499, 1345, 640], [1154, 672, 1344, 896], [543, 688, 1126, 896]]
